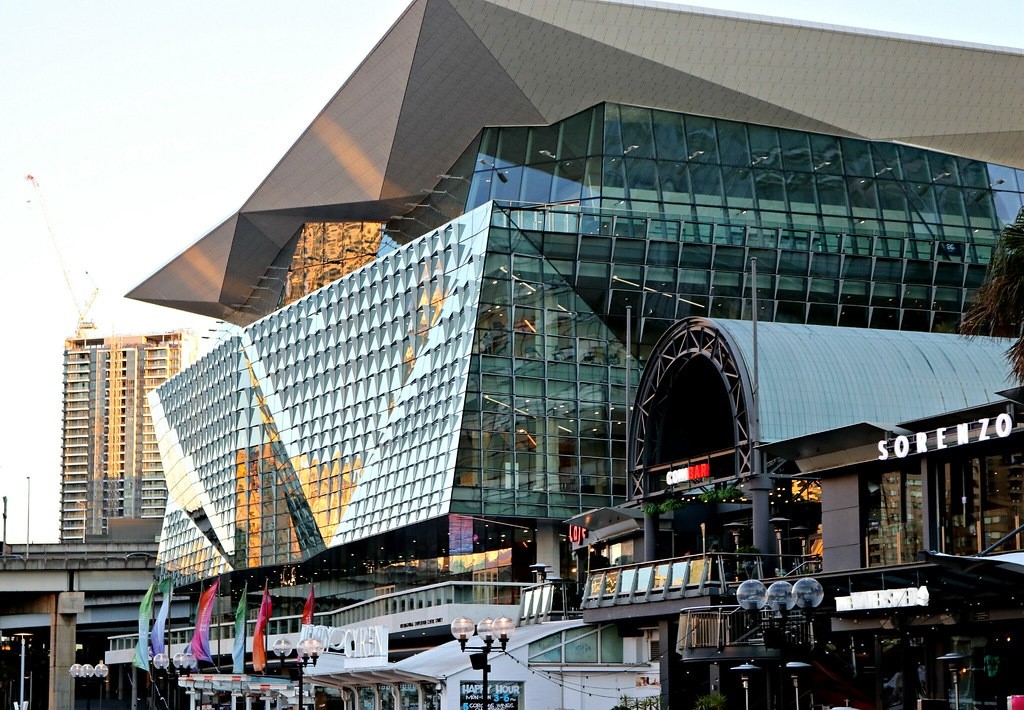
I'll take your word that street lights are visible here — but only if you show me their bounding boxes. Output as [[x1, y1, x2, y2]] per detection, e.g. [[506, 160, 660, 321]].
[[273, 638, 321, 710], [152, 653, 198, 710], [69, 663, 109, 710], [14, 633, 35, 710], [778, 661, 813, 710], [729, 663, 761, 710], [937, 652, 971, 710], [737, 576, 825, 710], [451, 614, 517, 710]]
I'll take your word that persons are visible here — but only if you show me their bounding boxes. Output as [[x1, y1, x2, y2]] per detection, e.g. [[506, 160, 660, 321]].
[[680, 550, 700, 562], [706, 544, 719, 565]]
